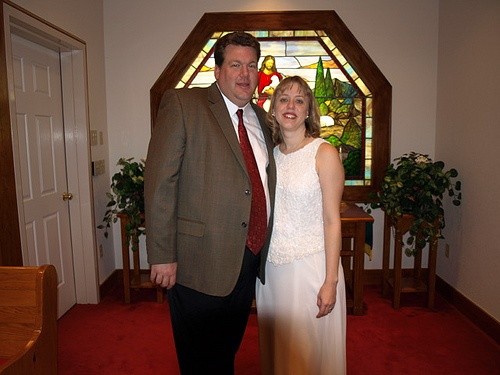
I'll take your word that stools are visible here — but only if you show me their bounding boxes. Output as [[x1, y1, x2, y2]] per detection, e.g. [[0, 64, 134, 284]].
[[116, 209, 164, 304], [382, 211, 444, 308]]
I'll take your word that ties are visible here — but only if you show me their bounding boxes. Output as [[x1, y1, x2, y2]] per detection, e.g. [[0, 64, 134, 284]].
[[236, 109, 268, 255]]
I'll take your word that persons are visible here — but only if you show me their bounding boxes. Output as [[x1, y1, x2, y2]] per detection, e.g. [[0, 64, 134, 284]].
[[255, 76, 347, 375], [143, 32, 277, 375]]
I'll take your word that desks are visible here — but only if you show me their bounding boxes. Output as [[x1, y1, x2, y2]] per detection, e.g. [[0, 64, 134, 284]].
[[341, 203, 374, 314]]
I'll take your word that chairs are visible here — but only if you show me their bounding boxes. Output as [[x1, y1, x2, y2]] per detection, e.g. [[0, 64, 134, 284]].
[[0, 264, 58, 375]]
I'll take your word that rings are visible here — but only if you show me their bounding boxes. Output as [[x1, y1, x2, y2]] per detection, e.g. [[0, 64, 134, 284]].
[[329, 308, 333, 310]]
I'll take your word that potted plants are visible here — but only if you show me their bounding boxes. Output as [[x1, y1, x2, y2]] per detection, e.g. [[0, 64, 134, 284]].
[[366, 151, 462, 257], [98, 157, 147, 243]]
